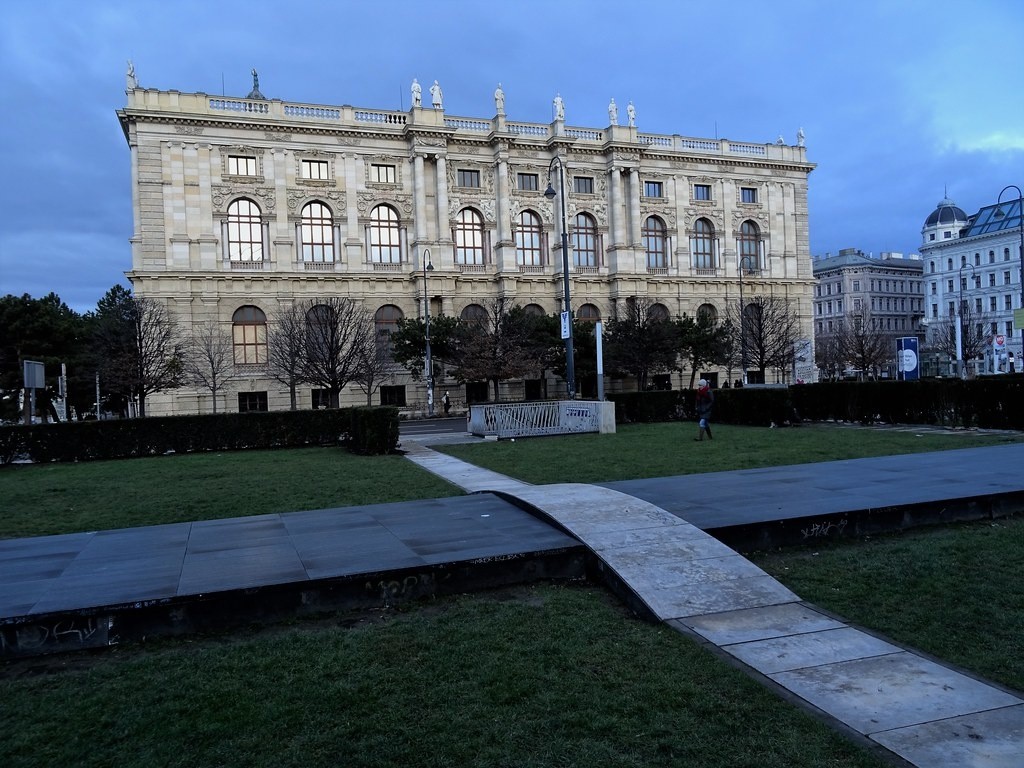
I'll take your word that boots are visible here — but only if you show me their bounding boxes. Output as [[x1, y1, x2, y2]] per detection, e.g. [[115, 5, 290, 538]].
[[694, 427, 706, 441]]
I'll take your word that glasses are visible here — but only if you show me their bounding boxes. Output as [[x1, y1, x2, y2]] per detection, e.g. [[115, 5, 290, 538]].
[[706, 427, 712, 439], [699, 384, 702, 386]]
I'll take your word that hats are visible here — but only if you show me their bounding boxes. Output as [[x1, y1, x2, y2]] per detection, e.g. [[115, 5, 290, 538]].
[[698, 379, 706, 387]]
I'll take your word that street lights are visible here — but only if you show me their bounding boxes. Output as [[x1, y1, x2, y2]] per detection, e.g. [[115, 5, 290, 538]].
[[994, 185, 1024, 372], [739, 256, 760, 385], [959, 263, 978, 361], [544, 154, 576, 402], [422, 248, 435, 419]]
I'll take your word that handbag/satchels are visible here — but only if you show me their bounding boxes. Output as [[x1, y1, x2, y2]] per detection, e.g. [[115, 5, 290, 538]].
[[442, 396, 446, 403]]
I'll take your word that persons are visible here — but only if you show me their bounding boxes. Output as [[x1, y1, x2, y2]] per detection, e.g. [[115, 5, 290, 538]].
[[694, 378, 715, 442], [126, 59, 805, 147], [722, 379, 743, 388], [664, 380, 673, 391], [441, 390, 452, 414]]
